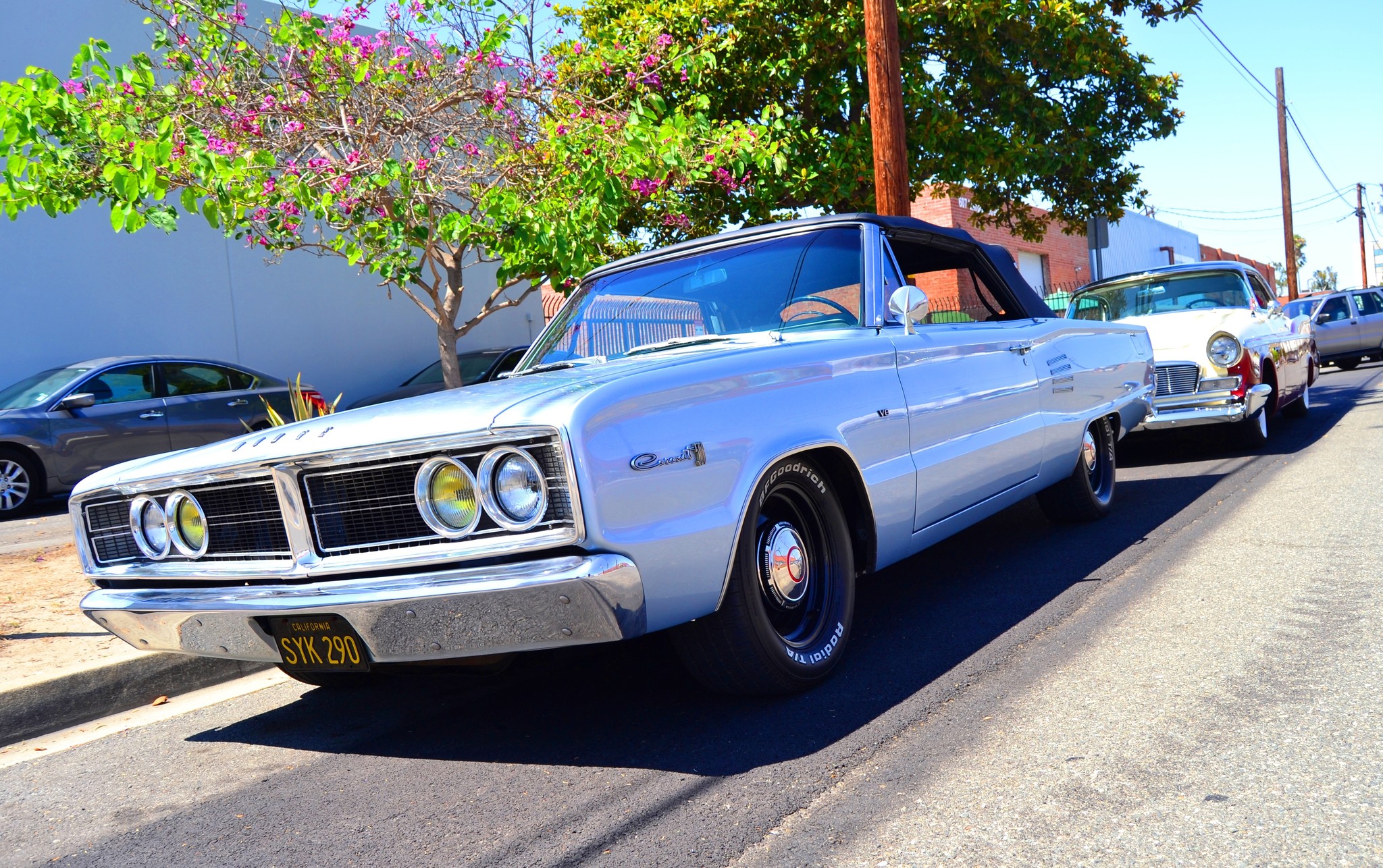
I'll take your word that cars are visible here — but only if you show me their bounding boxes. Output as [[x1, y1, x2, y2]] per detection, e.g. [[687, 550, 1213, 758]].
[[0, 354, 332, 519], [1061, 260, 1321, 447], [345, 345, 585, 410], [64, 210, 1156, 708]]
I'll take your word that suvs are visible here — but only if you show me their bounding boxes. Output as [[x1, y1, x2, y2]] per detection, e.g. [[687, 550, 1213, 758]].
[[1279, 285, 1383, 370]]
